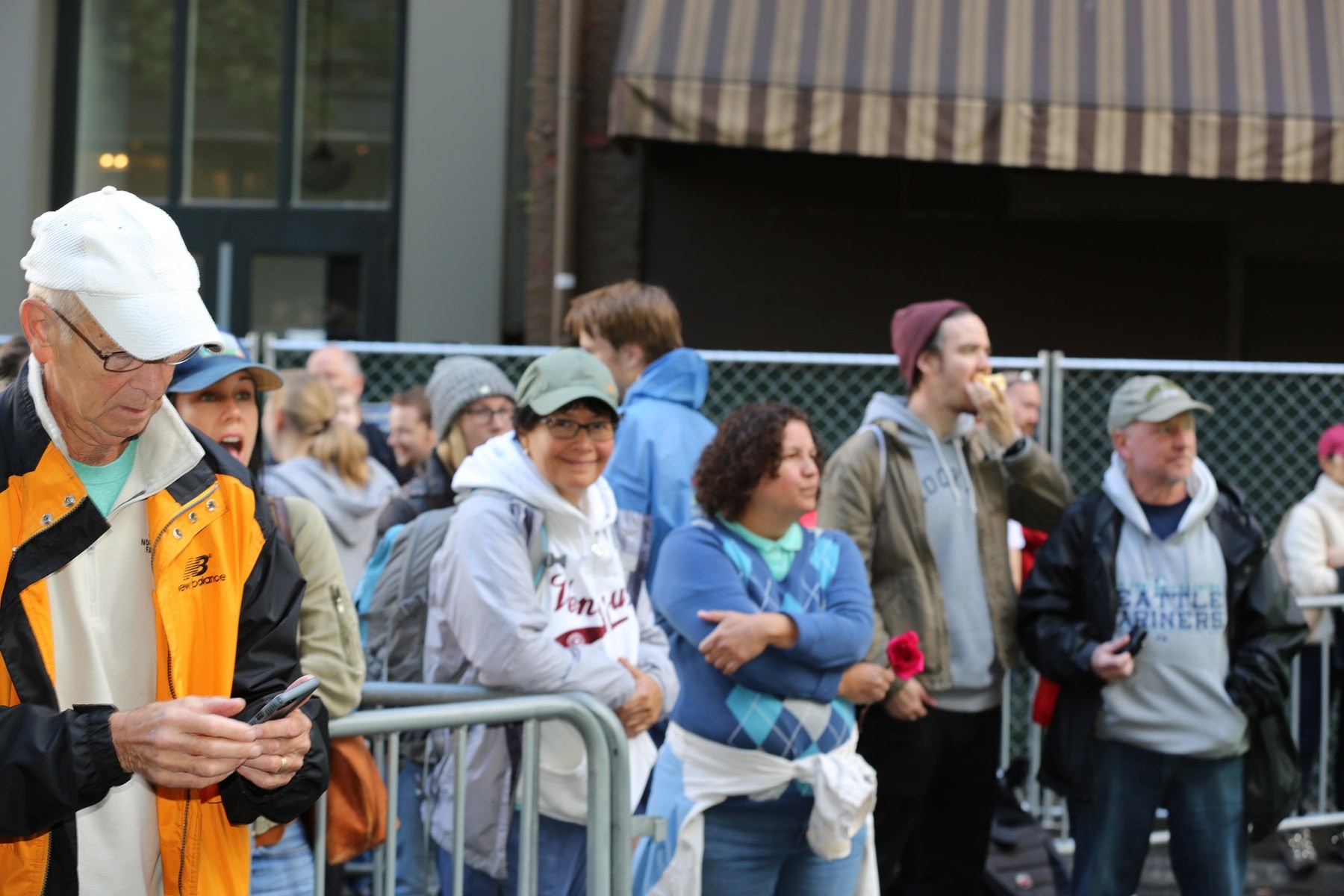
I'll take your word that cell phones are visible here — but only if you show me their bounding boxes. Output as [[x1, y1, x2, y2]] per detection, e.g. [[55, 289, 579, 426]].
[[246, 679, 320, 726]]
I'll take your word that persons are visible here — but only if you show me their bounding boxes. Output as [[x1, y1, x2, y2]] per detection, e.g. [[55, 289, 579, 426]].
[[0, 183, 331, 896], [0, 280, 1344, 896]]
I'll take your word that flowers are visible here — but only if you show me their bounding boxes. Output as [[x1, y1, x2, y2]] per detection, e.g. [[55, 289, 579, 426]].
[[859, 631, 923, 724]]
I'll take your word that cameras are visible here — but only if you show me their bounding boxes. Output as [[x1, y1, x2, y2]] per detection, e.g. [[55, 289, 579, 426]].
[[1116, 625, 1147, 655]]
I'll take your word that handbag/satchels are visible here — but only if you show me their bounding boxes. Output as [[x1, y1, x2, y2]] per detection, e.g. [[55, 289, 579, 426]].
[[323, 735, 400, 866]]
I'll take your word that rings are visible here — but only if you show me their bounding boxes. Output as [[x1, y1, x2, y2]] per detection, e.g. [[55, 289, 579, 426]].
[[275, 755, 287, 775]]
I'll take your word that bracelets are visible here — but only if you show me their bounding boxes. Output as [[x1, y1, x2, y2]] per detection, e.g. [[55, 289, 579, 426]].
[[1001, 437, 1025, 457]]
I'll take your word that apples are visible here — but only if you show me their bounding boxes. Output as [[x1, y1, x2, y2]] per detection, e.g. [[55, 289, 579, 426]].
[[972, 371, 1009, 399]]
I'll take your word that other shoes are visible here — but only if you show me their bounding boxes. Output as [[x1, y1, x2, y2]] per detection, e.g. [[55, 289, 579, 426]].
[[1279, 828, 1322, 874]]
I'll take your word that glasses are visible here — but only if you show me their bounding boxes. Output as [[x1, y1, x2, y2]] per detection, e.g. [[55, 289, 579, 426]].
[[538, 417, 616, 441], [33, 295, 202, 373], [461, 403, 513, 426]]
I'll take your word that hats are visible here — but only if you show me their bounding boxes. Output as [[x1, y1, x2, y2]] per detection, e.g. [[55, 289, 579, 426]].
[[1317, 423, 1344, 455], [513, 347, 620, 422], [1106, 374, 1213, 436], [19, 186, 226, 363], [165, 326, 284, 395], [890, 300, 969, 387], [423, 355, 517, 443]]
